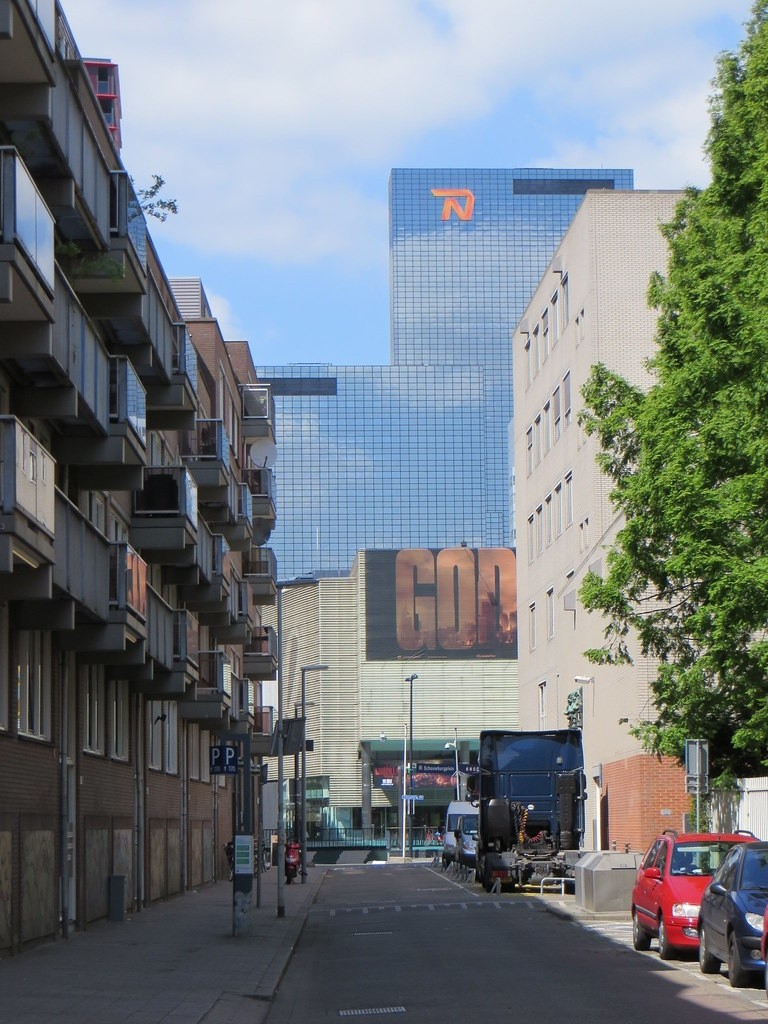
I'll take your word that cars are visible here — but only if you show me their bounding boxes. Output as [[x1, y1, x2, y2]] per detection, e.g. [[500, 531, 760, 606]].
[[696, 840, 768, 989], [632, 829, 760, 961]]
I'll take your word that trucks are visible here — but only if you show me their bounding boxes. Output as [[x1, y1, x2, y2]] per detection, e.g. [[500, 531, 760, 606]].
[[466, 729, 602, 893]]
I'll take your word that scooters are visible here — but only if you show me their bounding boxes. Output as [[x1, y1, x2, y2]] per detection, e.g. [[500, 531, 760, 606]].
[[285, 838, 304, 885]]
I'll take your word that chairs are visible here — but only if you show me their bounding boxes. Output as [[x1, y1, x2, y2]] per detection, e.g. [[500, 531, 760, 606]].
[[743, 860, 768, 885], [672, 846, 698, 871]]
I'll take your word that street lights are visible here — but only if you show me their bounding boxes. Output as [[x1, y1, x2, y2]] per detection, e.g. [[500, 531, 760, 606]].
[[408, 673, 419, 859], [445, 727, 460, 801], [294, 702, 316, 844], [272, 579, 323, 917], [300, 665, 330, 884]]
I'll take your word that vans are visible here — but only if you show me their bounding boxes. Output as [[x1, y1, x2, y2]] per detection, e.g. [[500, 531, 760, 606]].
[[439, 799, 480, 874]]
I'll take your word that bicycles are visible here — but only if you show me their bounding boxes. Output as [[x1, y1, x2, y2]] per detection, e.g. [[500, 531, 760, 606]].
[[258, 839, 271, 872], [224, 841, 235, 882]]
